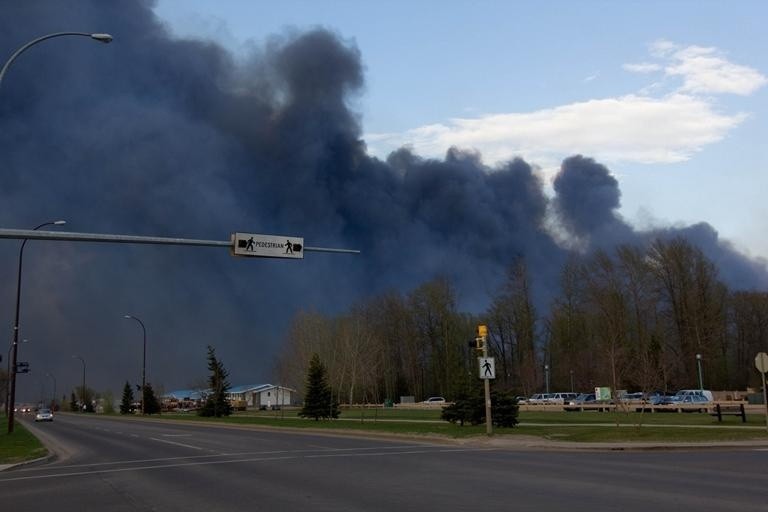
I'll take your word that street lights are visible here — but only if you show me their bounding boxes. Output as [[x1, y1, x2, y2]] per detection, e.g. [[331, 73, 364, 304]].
[[8, 218, 67, 433], [72, 355, 86, 403], [696, 354, 704, 389], [1, 32, 114, 83], [123, 314, 147, 417]]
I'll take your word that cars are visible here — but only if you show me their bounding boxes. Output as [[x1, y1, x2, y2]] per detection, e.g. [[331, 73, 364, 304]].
[[32, 409, 53, 421], [423, 396, 446, 405], [516, 389, 715, 413]]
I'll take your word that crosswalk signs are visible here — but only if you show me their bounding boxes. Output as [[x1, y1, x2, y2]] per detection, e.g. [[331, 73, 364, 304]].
[[478, 356, 495, 379]]
